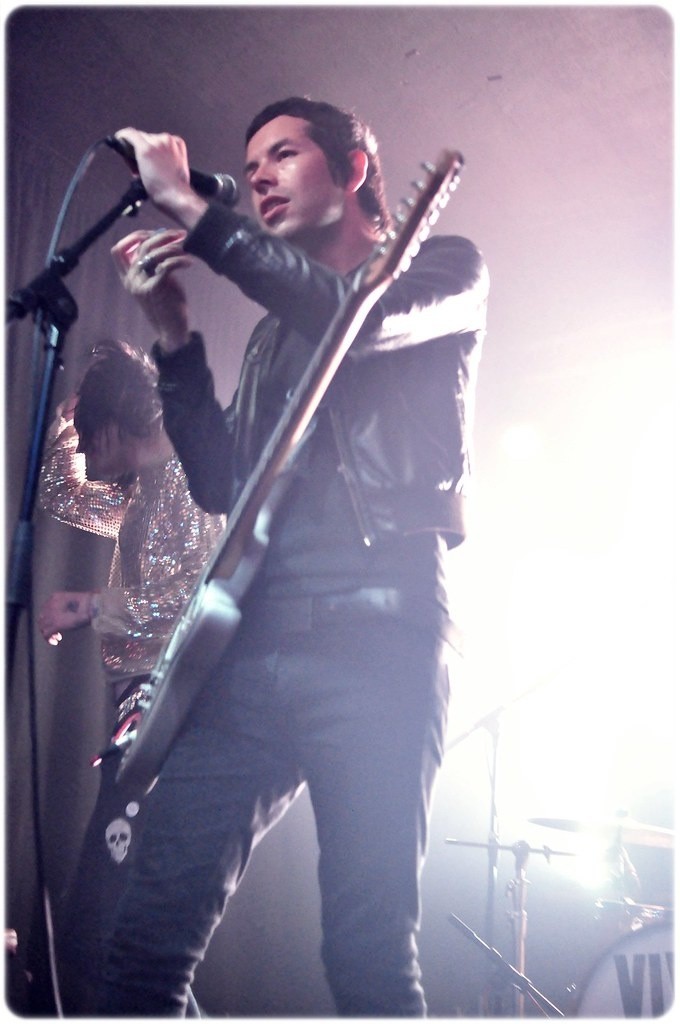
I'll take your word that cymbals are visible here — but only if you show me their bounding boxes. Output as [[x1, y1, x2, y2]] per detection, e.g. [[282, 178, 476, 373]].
[[527, 816, 676, 845]]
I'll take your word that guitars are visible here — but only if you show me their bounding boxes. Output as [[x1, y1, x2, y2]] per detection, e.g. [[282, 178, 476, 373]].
[[112, 146, 468, 800]]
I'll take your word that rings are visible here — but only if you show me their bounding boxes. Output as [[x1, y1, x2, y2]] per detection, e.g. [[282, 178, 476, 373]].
[[154, 263, 168, 278], [138, 256, 155, 270]]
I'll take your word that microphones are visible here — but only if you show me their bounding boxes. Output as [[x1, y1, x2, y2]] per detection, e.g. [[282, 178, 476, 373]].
[[105, 135, 241, 208]]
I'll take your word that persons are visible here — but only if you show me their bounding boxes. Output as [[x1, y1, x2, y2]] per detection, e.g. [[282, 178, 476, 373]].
[[38, 339, 227, 1018], [98, 95, 490, 1018]]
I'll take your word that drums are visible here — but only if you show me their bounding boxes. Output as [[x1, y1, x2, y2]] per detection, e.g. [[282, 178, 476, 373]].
[[571, 919, 674, 1017], [589, 899, 674, 945]]
